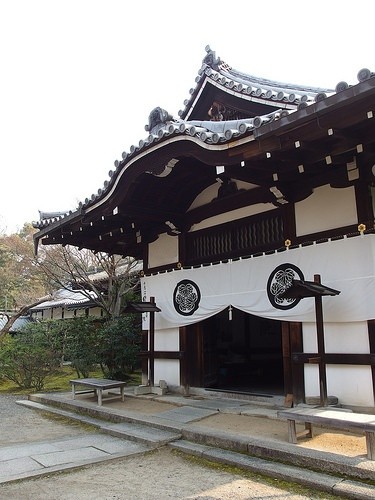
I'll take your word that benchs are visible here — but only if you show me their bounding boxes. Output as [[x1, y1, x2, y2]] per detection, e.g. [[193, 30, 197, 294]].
[[70, 377, 126, 406], [277, 407, 375, 462]]
